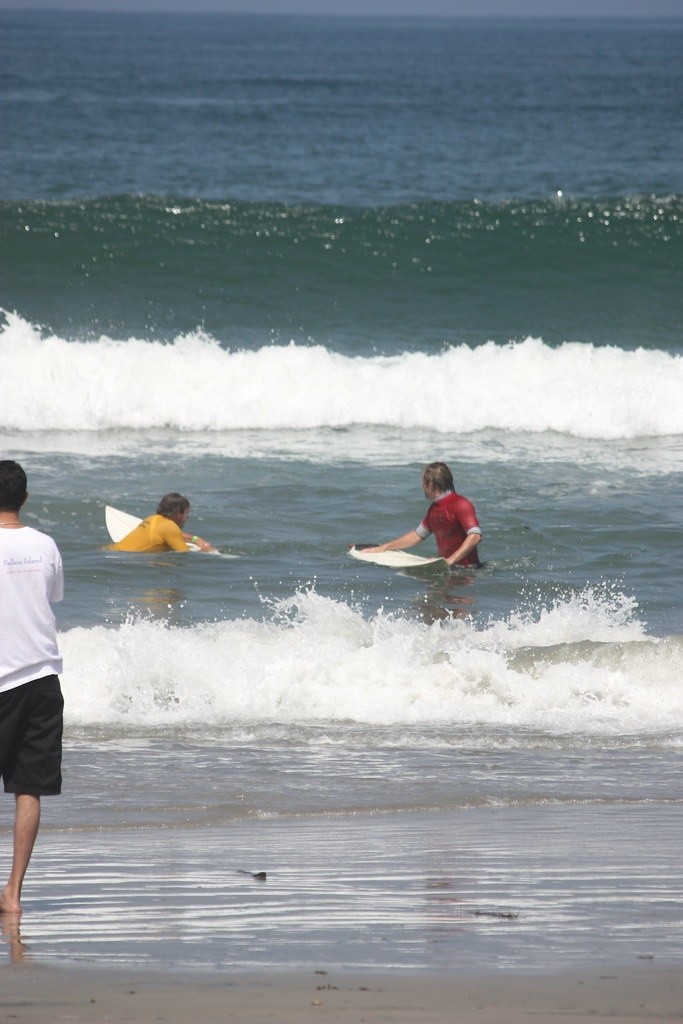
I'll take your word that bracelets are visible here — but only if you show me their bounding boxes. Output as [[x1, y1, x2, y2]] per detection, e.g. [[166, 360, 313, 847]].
[[192, 536, 197, 543]]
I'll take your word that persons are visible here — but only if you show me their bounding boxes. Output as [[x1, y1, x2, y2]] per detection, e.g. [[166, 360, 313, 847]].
[[113, 492, 208, 552], [373, 462, 481, 566], [0, 459, 64, 913]]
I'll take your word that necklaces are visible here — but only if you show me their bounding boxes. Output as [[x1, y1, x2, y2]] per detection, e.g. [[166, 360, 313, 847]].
[[0, 522, 21, 525]]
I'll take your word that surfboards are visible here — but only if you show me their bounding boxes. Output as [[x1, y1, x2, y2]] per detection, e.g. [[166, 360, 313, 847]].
[[351, 541, 442, 568], [101, 501, 216, 557]]
